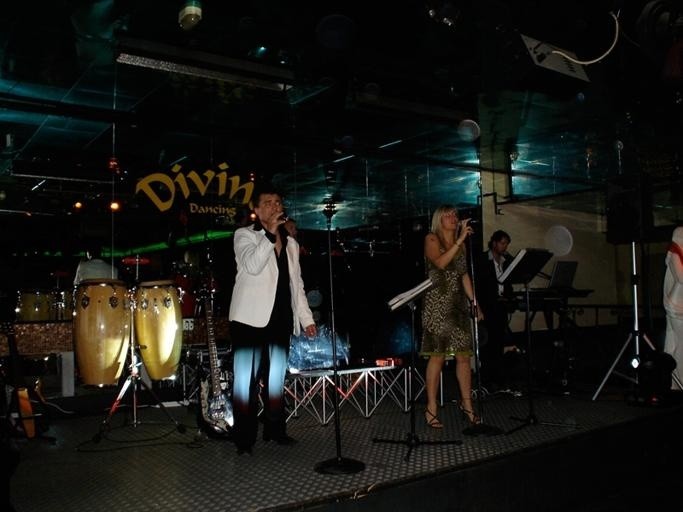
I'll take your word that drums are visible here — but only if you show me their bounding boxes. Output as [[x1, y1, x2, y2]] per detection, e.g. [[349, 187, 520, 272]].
[[135, 281, 182, 380], [73, 279, 131, 389], [15, 291, 73, 323]]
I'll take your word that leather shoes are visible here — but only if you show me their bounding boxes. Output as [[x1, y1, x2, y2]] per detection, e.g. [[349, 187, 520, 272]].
[[236, 445, 253, 457], [265, 435, 298, 445]]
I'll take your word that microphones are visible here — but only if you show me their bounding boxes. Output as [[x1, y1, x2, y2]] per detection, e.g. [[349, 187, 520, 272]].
[[457, 220, 479, 226], [272, 213, 288, 224]]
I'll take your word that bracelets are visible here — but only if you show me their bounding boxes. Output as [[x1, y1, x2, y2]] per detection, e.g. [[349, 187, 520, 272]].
[[453, 241, 461, 248]]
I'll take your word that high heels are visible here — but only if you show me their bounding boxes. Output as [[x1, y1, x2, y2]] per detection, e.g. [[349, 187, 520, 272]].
[[458, 398, 481, 425], [424, 406, 443, 428]]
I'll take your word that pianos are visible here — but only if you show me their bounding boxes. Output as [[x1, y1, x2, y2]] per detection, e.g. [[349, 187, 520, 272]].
[[490, 296, 563, 310]]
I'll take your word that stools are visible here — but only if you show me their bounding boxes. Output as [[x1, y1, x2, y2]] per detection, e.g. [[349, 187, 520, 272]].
[[158, 343, 443, 427]]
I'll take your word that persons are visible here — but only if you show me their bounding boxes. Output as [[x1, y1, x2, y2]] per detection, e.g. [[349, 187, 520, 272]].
[[73, 241, 118, 286], [467, 230, 516, 332], [663, 228, 683, 391], [183, 249, 206, 278], [229, 188, 317, 457], [419, 204, 484, 428]]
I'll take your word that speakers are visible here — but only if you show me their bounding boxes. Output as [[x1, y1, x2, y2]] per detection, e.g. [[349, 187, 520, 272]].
[[606, 177, 654, 244]]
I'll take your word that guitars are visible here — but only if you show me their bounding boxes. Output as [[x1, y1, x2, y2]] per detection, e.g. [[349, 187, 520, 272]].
[[1, 320, 49, 439], [193, 283, 235, 432]]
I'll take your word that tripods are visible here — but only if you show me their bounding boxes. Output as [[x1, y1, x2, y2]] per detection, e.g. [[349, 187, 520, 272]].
[[372, 303, 463, 462], [92, 289, 187, 446], [592, 240, 683, 401], [505, 279, 582, 435]]
